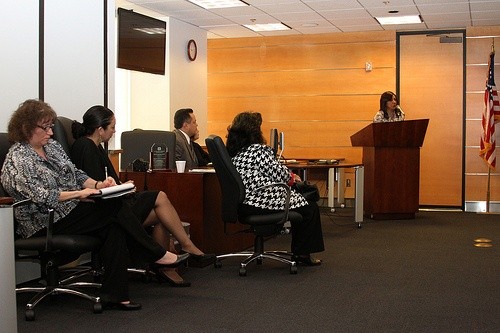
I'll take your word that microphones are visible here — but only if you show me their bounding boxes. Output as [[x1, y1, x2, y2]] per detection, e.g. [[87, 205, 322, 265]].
[[395, 105, 405, 115]]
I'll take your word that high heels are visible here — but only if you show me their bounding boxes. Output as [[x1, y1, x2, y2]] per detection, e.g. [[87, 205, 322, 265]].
[[156, 269, 191, 288], [180, 249, 216, 268], [291, 253, 321, 265]]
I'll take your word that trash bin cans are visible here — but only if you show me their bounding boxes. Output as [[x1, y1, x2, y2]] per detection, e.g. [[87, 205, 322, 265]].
[[151, 222, 190, 272]]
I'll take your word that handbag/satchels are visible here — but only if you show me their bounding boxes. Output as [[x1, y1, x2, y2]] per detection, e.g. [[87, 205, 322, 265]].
[[295, 182, 319, 201]]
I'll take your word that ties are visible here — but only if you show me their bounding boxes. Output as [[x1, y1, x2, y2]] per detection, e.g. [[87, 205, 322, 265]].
[[190, 140, 195, 160]]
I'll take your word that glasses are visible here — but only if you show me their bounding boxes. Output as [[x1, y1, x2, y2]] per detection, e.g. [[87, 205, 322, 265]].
[[32, 124, 55, 132]]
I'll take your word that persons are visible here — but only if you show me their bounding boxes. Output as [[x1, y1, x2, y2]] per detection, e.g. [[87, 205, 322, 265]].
[[192, 134, 211, 166], [173, 108, 198, 169], [227, 112, 325, 265], [71, 105, 217, 287], [374, 91, 405, 123], [0, 99, 190, 310]]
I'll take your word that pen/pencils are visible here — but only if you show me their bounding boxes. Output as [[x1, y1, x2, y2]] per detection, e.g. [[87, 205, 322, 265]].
[[105, 166, 108, 179]]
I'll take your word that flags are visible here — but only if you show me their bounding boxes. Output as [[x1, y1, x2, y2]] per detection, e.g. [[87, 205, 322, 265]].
[[479, 50, 500, 169]]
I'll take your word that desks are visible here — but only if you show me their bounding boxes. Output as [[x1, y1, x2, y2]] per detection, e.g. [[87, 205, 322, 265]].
[[119, 166, 255, 268], [287, 158, 365, 229]]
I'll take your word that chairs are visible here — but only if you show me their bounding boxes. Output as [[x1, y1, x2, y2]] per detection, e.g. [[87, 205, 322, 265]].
[[205, 135, 303, 276], [0, 117, 148, 322]]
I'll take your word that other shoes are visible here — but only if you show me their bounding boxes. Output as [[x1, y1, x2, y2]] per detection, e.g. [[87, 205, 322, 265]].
[[102, 296, 140, 310], [150, 252, 190, 271]]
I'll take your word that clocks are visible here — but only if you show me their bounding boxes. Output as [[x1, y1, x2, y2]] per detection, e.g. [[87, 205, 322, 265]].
[[187, 40, 198, 62]]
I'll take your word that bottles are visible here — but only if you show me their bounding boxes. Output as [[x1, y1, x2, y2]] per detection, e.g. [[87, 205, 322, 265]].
[[376, 110, 382, 122]]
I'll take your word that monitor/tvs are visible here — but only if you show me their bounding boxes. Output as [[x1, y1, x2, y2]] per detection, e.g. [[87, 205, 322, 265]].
[[116, 7, 166, 75], [270, 129, 284, 155]]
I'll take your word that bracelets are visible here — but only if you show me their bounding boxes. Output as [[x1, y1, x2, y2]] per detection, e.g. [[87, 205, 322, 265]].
[[95, 181, 98, 188]]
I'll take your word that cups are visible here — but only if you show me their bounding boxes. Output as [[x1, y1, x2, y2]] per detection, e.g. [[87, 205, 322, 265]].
[[175, 160, 187, 173]]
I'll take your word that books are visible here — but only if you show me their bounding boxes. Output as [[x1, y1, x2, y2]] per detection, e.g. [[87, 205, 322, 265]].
[[91, 182, 137, 199]]
